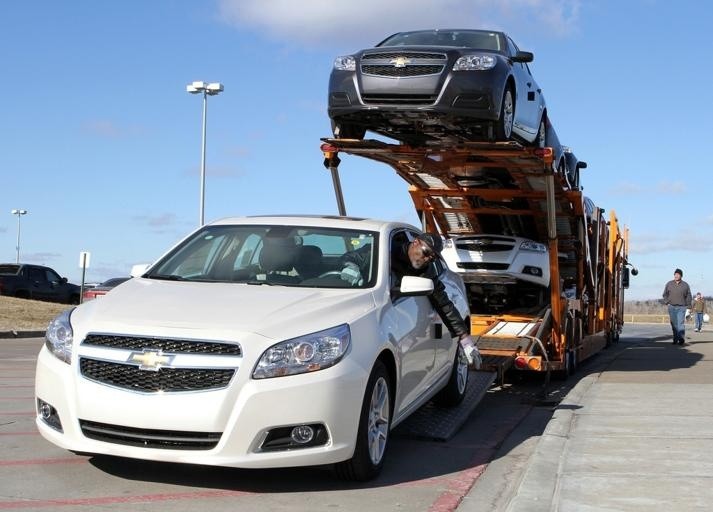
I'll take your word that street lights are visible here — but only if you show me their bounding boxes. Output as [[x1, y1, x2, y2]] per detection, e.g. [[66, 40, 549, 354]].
[[184, 77, 229, 231], [10, 209, 27, 264]]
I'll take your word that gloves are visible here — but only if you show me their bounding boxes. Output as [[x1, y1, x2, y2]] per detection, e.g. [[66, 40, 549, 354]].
[[457, 334, 483, 371]]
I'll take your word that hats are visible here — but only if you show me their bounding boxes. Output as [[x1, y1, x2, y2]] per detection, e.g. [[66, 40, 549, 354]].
[[417, 231, 447, 263], [674, 268, 682, 277]]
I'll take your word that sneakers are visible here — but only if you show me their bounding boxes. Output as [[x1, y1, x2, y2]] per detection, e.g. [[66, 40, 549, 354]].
[[699, 330, 703, 333], [693, 328, 699, 333]]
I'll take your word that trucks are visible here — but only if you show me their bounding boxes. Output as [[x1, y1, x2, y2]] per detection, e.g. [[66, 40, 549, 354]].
[[319, 138, 637, 443]]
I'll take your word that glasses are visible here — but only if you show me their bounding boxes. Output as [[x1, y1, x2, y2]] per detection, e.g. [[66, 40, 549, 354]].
[[417, 238, 435, 262]]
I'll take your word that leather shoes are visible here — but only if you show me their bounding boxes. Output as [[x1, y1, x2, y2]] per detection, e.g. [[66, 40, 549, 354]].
[[673, 340, 678, 344], [678, 340, 684, 345]]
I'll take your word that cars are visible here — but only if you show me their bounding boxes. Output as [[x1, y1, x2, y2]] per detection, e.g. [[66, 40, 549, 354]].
[[321, 20, 553, 159], [437, 122, 624, 323], [82, 277, 132, 305], [0, 261, 81, 307], [19, 205, 472, 488]]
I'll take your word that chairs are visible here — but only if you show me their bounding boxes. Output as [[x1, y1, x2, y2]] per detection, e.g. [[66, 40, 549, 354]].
[[246, 244, 299, 289]]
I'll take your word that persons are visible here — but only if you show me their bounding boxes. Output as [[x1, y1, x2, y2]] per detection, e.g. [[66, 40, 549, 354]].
[[662, 268, 692, 347], [336, 232, 483, 370], [687, 293, 706, 331]]
